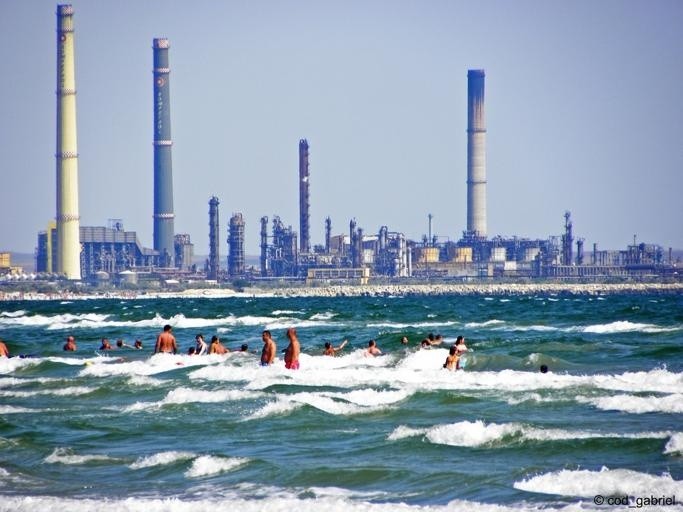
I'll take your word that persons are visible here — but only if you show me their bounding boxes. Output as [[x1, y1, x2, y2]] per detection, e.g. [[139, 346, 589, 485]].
[[284, 327, 300, 370], [63, 334, 469, 371], [260, 330, 276, 366], [154, 325, 176, 354], [539, 364, 553, 374], [0, 341, 8, 358]]
